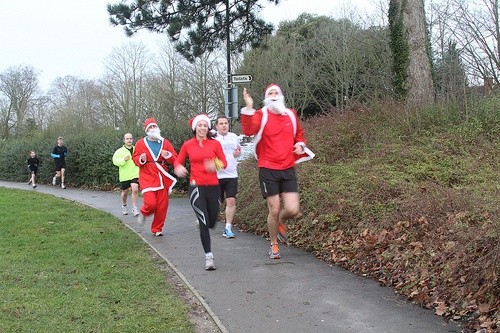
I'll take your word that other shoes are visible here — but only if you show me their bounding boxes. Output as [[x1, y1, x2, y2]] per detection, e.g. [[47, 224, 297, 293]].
[[52, 177, 57, 185], [28, 179, 32, 185], [60, 183, 66, 189], [32, 184, 37, 188]]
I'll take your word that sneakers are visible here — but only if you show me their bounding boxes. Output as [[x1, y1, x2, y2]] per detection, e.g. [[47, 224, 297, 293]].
[[222, 227, 235, 238], [277, 223, 287, 242], [137, 212, 145, 226], [268, 243, 281, 259], [151, 231, 163, 236], [205, 253, 216, 270], [131, 207, 139, 216], [121, 205, 128, 214]]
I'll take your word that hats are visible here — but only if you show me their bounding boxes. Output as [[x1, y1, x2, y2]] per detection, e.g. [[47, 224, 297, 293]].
[[143, 118, 158, 133], [189, 114, 211, 131], [265, 83, 282, 99]]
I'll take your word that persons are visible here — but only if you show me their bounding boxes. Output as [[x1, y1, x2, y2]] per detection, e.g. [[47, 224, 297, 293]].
[[241, 84, 315, 259], [26, 150, 40, 188], [172, 114, 228, 270], [112, 133, 140, 217], [50, 137, 68, 189], [210, 116, 242, 239], [132, 118, 180, 237]]
